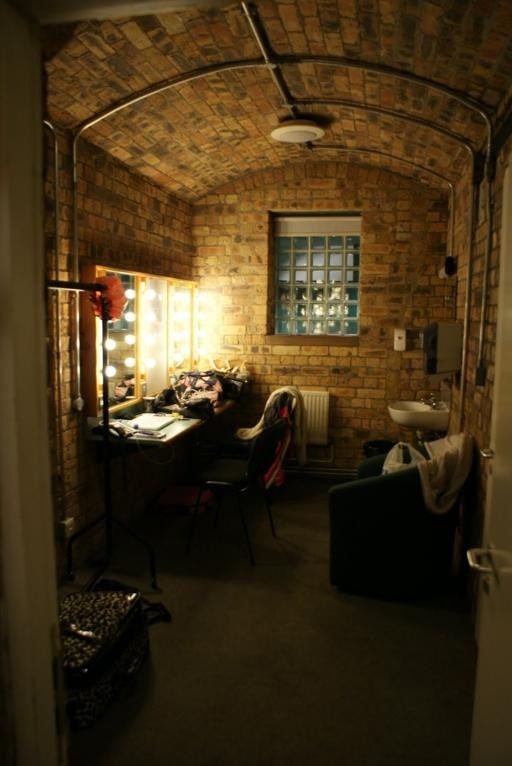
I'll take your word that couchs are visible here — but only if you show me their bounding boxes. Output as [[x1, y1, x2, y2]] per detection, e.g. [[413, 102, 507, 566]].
[[325, 433, 482, 610]]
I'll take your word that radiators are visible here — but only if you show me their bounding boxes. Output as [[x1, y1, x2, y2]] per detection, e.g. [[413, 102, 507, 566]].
[[290, 390, 331, 446]]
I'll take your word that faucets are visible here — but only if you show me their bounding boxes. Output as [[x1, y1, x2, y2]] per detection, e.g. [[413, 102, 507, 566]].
[[421, 392, 440, 407]]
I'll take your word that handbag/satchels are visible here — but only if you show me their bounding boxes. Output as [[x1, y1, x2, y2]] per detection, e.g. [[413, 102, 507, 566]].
[[115, 374, 135, 397], [174, 370, 227, 407]]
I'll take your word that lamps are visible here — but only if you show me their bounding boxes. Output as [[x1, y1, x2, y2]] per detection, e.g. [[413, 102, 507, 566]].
[[268, 117, 326, 144]]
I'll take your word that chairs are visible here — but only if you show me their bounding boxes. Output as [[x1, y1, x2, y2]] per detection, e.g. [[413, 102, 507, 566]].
[[177, 417, 288, 568], [231, 384, 305, 485]]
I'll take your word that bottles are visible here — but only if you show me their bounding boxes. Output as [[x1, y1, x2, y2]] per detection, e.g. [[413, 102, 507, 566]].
[[167, 366, 177, 386], [139, 372, 147, 397], [97, 384, 105, 412]]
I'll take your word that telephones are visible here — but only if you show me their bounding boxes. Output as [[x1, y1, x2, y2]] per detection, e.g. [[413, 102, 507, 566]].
[[100, 418, 135, 436]]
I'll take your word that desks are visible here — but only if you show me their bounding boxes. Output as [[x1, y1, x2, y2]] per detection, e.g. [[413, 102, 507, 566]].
[[161, 385, 238, 480], [88, 411, 207, 547]]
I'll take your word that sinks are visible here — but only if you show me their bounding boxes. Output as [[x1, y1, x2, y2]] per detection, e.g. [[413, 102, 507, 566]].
[[387, 401, 450, 432]]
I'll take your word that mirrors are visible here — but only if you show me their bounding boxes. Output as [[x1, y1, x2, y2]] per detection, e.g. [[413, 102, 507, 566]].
[[80, 261, 198, 418]]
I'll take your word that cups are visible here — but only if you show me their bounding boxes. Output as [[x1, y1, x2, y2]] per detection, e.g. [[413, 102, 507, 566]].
[[142, 396, 156, 412]]
